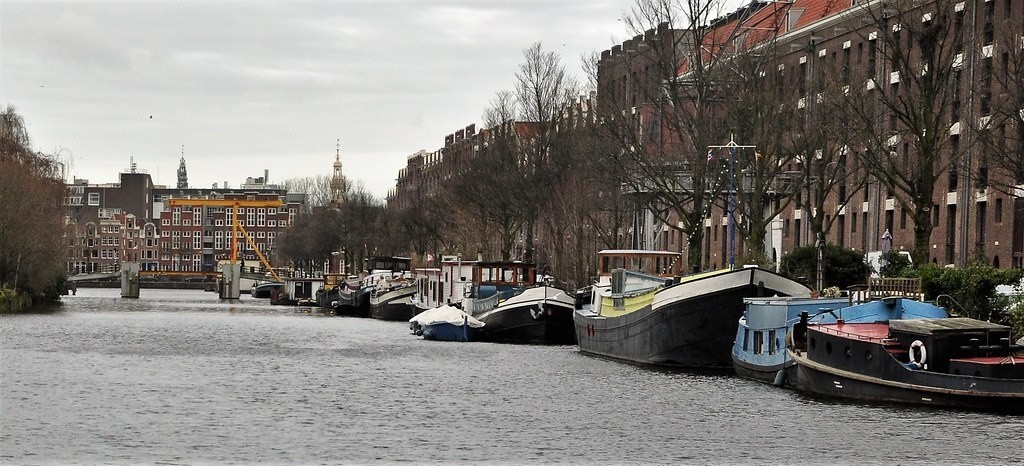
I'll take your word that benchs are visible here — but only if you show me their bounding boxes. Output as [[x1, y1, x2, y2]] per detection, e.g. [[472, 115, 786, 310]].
[[868, 276, 921, 302]]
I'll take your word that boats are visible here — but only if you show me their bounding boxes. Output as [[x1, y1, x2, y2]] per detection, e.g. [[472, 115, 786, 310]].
[[572, 133, 810, 370], [728, 274, 950, 387], [339, 242, 411, 318], [270, 292, 300, 305], [409, 250, 575, 345], [785, 308, 1024, 411], [410, 304, 487, 341], [299, 299, 317, 306], [370, 266, 429, 320], [315, 272, 338, 308], [251, 280, 284, 299]]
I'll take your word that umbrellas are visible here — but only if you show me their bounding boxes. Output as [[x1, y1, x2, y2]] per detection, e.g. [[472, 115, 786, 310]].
[[881, 228, 893, 268]]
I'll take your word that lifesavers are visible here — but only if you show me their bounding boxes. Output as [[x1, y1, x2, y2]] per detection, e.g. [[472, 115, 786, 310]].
[[908, 339, 927, 368]]
